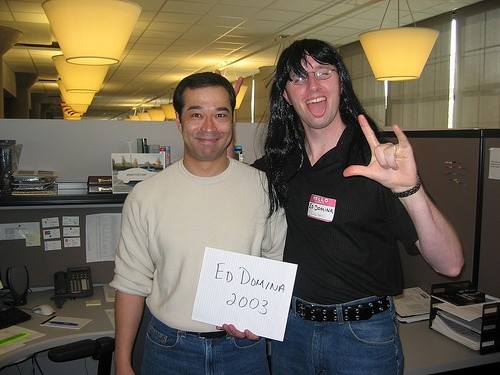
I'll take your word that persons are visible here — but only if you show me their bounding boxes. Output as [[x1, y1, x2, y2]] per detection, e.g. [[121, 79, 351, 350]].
[[247, 38, 465, 375], [106, 72, 287, 375]]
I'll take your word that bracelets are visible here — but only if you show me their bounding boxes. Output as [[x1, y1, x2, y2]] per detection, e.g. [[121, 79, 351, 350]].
[[392, 174, 421, 197]]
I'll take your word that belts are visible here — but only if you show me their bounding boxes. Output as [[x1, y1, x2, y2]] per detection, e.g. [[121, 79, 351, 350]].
[[291, 296, 391, 322], [190, 332, 225, 340]]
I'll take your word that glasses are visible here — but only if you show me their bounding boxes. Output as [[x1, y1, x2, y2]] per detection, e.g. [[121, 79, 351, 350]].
[[289, 69, 339, 84]]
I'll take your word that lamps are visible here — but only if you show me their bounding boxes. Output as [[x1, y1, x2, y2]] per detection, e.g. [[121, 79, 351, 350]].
[[358, 0, 440, 81], [41, 0, 248, 120]]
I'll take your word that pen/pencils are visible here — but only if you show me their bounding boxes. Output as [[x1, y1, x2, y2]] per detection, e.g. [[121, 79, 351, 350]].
[[49, 321, 78, 325]]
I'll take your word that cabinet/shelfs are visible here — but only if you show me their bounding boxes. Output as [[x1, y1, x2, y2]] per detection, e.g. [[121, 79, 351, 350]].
[[0, 183, 131, 375]]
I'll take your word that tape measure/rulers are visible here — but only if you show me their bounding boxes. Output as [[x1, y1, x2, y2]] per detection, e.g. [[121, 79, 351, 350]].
[[0, 332, 28, 344]]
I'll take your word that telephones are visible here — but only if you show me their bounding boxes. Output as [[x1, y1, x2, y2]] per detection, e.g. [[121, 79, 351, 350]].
[[54, 267, 94, 300]]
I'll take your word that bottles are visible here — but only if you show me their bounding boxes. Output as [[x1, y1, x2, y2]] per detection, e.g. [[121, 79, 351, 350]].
[[234, 146, 243, 162]]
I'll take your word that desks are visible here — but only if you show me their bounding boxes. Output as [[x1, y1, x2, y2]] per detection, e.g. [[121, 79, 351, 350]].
[[399, 320, 500, 375]]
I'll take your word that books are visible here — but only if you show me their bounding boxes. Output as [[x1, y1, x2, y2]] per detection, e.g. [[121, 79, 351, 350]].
[[87, 175, 112, 193], [392, 286, 431, 323], [0, 139, 58, 196], [431, 291, 500, 353]]
